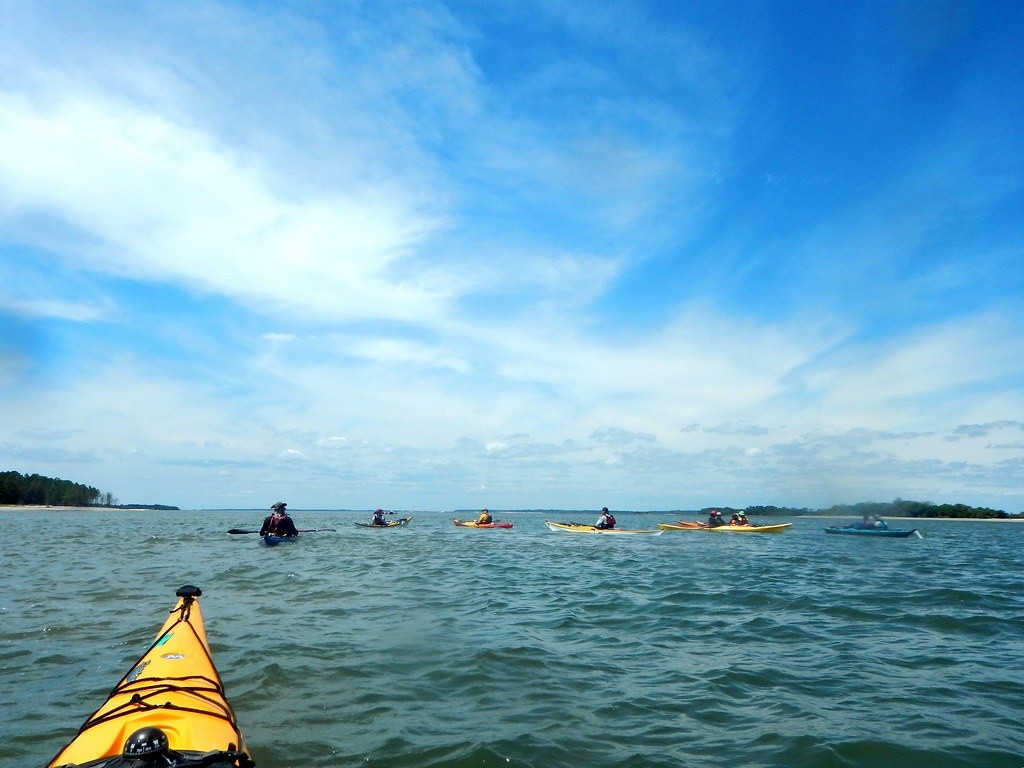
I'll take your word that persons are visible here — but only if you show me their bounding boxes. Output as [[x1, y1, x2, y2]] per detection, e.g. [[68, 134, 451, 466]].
[[372, 509, 386, 525], [259, 502, 298, 537], [729, 511, 748, 525], [716, 512, 725, 523], [708, 510, 723, 526], [474, 509, 489, 524], [863, 513, 887, 530], [596, 507, 615, 528]]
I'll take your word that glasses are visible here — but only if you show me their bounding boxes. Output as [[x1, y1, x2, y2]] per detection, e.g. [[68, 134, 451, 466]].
[[281, 507, 286, 510]]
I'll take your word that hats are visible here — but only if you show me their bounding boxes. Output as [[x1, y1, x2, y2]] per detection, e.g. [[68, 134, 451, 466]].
[[374, 509, 383, 514], [731, 513, 738, 517], [271, 502, 287, 510], [481, 509, 488, 513], [711, 510, 716, 515], [739, 511, 745, 516], [717, 511, 722, 516]]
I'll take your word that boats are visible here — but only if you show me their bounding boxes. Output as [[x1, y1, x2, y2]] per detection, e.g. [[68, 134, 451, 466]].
[[545, 520, 665, 536], [453, 519, 513, 528], [824, 525, 916, 538], [265, 533, 297, 546], [355, 516, 413, 528], [45, 586, 257, 768], [657, 521, 792, 532]]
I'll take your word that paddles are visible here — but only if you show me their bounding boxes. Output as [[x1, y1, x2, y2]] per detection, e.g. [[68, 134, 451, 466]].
[[458, 518, 501, 525], [569, 521, 595, 527], [227, 527, 337, 534]]
